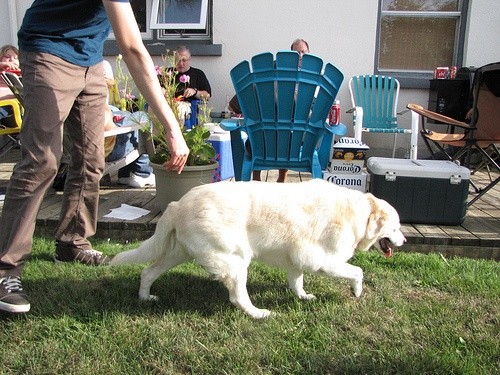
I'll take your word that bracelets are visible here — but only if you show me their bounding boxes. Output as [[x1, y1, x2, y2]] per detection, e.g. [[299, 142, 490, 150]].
[[192, 88, 198, 97]]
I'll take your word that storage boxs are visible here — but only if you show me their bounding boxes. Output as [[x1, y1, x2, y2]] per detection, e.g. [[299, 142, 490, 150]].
[[184, 123, 248, 183], [323, 136, 369, 193], [366, 156, 470, 226], [433, 66, 450, 79]]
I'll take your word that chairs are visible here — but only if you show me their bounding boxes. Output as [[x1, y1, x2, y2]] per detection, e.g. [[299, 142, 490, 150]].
[[220, 49, 500, 210], [0, 68, 207, 186]]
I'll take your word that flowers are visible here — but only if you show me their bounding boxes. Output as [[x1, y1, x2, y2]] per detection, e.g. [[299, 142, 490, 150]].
[[111, 48, 216, 165]]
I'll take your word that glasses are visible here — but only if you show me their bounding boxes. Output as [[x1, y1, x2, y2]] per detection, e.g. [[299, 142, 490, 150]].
[[176, 57, 191, 62]]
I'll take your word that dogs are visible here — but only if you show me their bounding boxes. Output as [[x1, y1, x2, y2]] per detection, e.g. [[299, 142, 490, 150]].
[[105, 179, 409, 320]]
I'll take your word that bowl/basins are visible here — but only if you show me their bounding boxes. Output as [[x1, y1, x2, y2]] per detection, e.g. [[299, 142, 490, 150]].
[[211, 111, 222, 118]]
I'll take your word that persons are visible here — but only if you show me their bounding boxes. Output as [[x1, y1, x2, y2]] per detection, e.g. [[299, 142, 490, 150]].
[[157, 46, 212, 128], [103, 87, 156, 188], [0, 45, 21, 71], [228, 39, 310, 183], [0, 0, 190, 313]]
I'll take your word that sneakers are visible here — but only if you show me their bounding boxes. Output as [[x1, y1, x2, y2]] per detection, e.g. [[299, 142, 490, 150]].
[[54, 244, 115, 266], [118, 169, 129, 185], [0, 276, 30, 313], [129, 171, 156, 188]]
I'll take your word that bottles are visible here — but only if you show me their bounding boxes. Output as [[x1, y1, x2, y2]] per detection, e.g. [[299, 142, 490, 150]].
[[224, 95, 231, 119]]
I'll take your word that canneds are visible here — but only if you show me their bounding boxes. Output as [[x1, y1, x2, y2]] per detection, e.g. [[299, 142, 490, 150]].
[[448, 65, 457, 80], [329, 100, 340, 126], [113, 114, 122, 123]]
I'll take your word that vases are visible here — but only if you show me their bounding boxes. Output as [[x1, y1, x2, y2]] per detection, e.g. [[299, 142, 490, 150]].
[[148, 154, 218, 216]]
